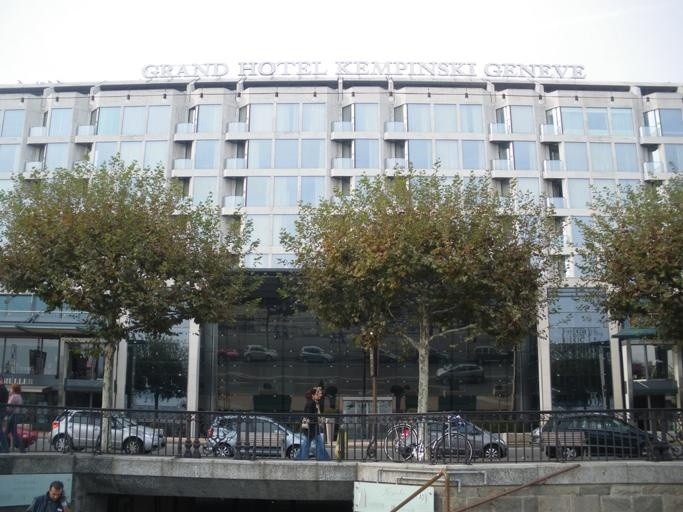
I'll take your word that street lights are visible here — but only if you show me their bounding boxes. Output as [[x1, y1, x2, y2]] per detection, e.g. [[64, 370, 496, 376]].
[[446, 332, 456, 408]]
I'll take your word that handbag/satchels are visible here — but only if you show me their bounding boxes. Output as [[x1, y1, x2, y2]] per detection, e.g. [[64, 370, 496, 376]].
[[301, 415, 310, 430]]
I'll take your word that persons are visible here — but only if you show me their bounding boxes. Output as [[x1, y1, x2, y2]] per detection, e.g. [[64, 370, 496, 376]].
[[0, 382, 26, 452], [295, 388, 330, 461], [312, 385, 324, 443], [24, 480, 71, 512], [0, 372, 8, 424]]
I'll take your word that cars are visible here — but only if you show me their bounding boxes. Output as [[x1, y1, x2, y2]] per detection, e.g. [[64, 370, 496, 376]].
[[416, 329, 509, 385], [259, 320, 361, 342], [202, 414, 315, 459], [526, 410, 674, 461], [298, 346, 335, 364], [392, 414, 507, 463], [199, 343, 278, 363], [379, 346, 401, 363], [46, 408, 165, 454]]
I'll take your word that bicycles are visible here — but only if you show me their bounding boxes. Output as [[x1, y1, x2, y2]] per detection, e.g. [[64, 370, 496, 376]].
[[383, 407, 473, 464]]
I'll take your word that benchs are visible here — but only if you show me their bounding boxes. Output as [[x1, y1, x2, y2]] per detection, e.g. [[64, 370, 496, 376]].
[[541, 431, 588, 459], [241, 432, 287, 459]]
